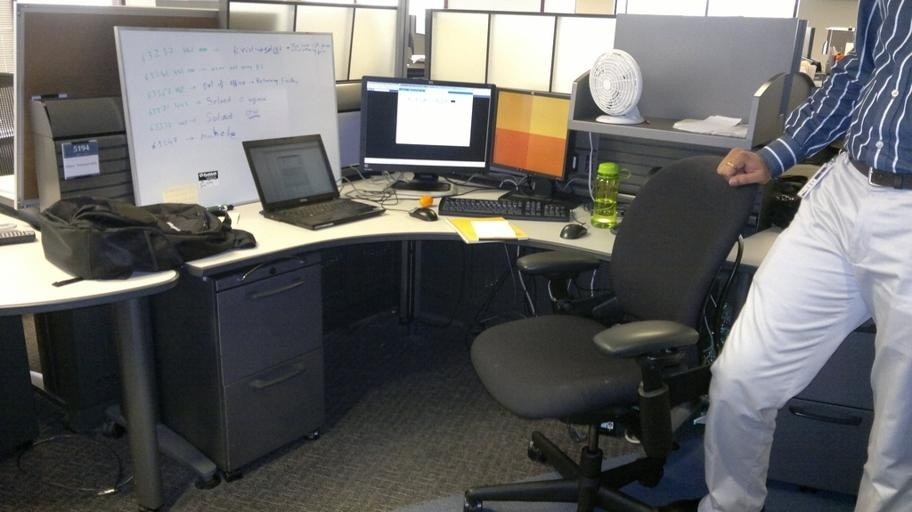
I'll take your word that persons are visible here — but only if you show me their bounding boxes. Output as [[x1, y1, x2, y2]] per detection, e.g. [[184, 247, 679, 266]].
[[692, 0, 912, 512]]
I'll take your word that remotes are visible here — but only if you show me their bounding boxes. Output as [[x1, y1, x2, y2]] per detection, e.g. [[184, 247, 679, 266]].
[[0, 230, 36, 245]]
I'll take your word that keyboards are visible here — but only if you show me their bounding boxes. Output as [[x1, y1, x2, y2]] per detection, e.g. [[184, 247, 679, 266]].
[[438, 196, 571, 222]]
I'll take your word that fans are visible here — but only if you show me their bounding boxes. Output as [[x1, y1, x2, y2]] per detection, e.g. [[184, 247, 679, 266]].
[[588, 48, 645, 124]]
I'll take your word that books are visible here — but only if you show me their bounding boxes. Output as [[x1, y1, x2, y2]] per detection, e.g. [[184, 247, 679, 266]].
[[471, 220, 519, 241], [445, 214, 530, 246]]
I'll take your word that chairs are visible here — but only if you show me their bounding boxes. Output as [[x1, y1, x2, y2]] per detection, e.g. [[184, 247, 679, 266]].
[[463, 154, 759, 512]]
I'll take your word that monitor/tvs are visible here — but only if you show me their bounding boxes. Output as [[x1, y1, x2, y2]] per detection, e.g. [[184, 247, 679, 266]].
[[359, 75, 496, 196], [490, 86, 582, 209]]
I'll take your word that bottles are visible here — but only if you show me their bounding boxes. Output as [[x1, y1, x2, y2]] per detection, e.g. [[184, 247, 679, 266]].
[[591, 162, 632, 229]]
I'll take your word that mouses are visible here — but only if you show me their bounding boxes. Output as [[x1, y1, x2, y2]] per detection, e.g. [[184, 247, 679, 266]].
[[560, 224, 587, 239], [409, 207, 437, 221]]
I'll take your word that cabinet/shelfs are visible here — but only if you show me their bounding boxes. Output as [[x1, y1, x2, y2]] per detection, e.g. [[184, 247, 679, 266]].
[[766, 318, 878, 504], [562, 13, 816, 229]]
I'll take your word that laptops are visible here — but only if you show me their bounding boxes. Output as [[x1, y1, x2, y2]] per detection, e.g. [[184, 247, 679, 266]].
[[242, 134, 387, 231]]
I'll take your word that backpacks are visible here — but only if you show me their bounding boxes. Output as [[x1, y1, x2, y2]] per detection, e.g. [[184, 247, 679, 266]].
[[41, 195, 256, 280]]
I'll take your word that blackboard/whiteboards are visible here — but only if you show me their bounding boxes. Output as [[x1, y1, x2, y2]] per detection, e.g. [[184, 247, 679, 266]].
[[113, 25, 343, 208]]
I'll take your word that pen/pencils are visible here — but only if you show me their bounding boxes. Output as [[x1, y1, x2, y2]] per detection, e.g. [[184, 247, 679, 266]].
[[32, 93, 69, 100]]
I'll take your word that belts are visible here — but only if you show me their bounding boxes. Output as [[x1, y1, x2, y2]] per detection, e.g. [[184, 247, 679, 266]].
[[848, 156, 912, 188]]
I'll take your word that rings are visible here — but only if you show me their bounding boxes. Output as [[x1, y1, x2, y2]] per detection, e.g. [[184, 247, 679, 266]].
[[726, 162, 739, 169]]
